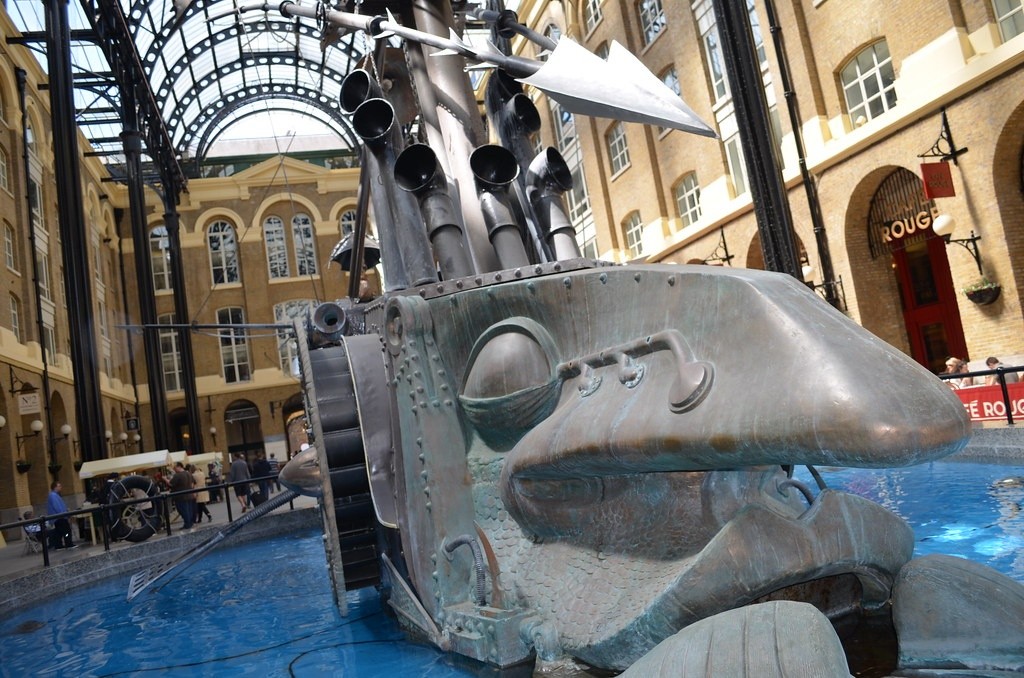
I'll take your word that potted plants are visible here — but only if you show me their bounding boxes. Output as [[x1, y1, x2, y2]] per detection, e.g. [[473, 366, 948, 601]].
[[16, 459, 32, 473], [74, 460, 83, 471], [48, 460, 63, 473]]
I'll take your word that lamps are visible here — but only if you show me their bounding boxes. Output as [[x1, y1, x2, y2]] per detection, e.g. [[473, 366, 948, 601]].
[[930, 215, 984, 276], [111, 432, 128, 450], [45, 424, 71, 456], [802, 266, 847, 312], [209, 426, 218, 447], [0, 415, 7, 433], [106, 431, 113, 444], [666, 229, 731, 267], [125, 434, 142, 454], [16, 420, 42, 457]]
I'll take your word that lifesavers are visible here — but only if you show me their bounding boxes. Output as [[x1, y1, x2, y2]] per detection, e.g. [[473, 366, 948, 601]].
[[106, 474, 166, 543]]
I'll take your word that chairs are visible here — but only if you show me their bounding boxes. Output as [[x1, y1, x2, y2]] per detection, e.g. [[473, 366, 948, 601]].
[[16, 517, 45, 555]]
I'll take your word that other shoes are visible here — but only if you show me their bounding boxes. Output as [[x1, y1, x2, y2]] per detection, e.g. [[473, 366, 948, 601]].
[[196, 520, 201, 524], [241, 506, 247, 514], [208, 518, 212, 523], [66, 544, 79, 550], [180, 525, 189, 530], [56, 545, 65, 550]]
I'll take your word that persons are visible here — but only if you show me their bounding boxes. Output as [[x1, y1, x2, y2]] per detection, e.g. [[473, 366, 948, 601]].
[[206, 464, 223, 503], [944, 357, 971, 388], [100, 472, 125, 544], [23, 511, 56, 549], [47, 481, 78, 550], [230, 453, 251, 512], [253, 452, 281, 498], [986, 357, 1019, 385], [142, 462, 212, 530]]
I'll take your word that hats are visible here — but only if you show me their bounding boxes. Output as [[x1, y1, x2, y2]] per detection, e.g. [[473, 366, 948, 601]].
[[946, 357, 961, 367]]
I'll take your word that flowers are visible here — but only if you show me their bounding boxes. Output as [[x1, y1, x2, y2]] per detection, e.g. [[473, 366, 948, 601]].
[[958, 278, 1002, 297]]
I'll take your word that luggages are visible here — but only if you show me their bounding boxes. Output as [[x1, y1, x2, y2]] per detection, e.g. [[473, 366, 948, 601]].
[[248, 485, 266, 507]]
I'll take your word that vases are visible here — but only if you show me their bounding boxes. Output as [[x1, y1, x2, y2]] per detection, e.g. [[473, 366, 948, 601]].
[[966, 287, 1000, 306]]
